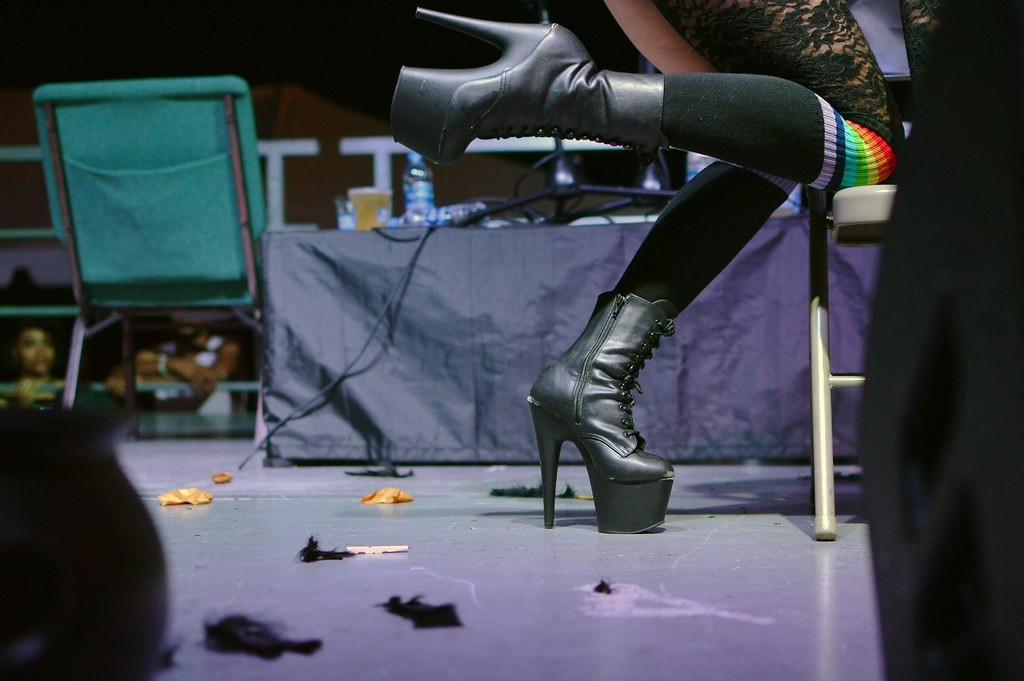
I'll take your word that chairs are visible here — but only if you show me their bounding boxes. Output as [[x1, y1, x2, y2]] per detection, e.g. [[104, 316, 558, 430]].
[[34, 76, 268, 442], [810, 76, 915, 539]]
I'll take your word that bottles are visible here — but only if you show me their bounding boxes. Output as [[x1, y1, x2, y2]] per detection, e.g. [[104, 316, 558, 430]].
[[389, 150, 490, 229]]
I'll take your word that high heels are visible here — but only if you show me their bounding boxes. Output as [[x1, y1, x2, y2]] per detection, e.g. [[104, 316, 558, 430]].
[[528, 291, 674, 533], [388, 6, 665, 165]]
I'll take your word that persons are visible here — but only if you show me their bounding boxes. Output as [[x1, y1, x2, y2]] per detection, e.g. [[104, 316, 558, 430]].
[[390, 0, 905, 535], [0, 402, 168, 681], [106, 308, 241, 439], [0, 325, 64, 412]]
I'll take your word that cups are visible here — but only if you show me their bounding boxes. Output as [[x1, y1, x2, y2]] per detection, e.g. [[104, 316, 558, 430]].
[[346, 187, 392, 230]]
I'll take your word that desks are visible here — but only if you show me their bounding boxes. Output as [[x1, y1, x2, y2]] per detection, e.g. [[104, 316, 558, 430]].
[[258, 212, 883, 469]]
